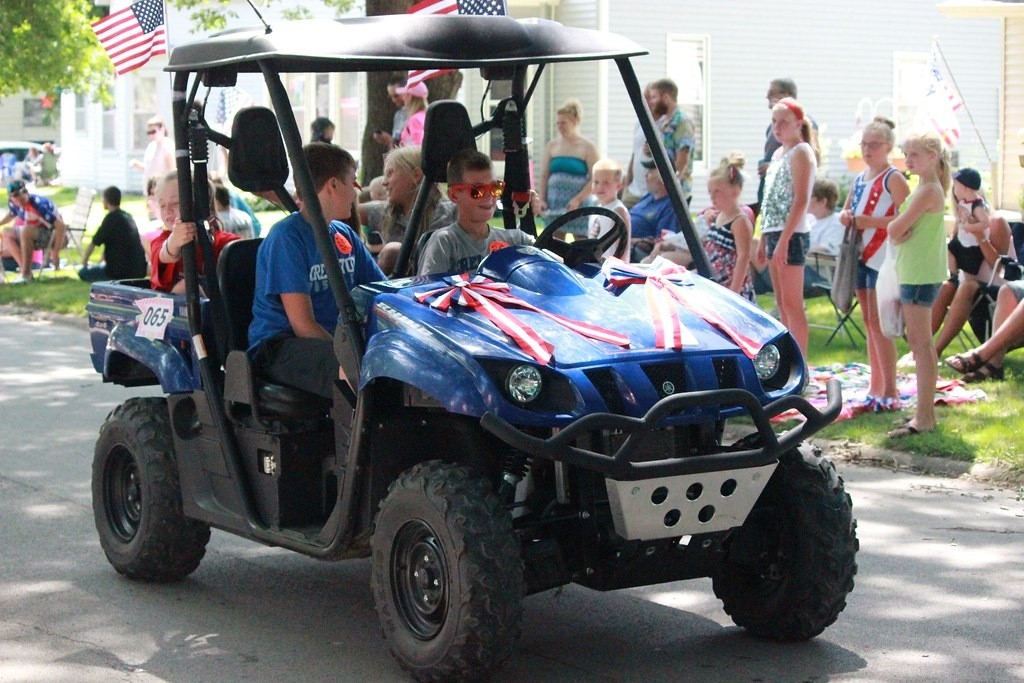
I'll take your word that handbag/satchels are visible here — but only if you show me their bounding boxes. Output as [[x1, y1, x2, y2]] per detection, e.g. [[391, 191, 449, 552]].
[[876, 236, 905, 337], [830, 217, 857, 313]]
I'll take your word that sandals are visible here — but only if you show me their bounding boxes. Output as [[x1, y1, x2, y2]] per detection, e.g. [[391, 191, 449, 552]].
[[945, 349, 982, 373], [963, 361, 1004, 380]]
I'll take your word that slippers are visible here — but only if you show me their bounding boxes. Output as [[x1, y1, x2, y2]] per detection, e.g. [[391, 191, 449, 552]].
[[894, 417, 913, 423], [888, 424, 918, 437]]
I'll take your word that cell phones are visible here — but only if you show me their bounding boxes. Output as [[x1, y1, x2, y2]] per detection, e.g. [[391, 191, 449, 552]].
[[374, 130, 381, 134]]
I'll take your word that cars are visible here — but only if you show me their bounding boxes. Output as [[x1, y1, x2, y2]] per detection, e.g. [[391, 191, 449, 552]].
[[0, 141, 44, 184]]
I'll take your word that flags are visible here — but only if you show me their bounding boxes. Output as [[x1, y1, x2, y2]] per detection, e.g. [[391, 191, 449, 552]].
[[405, 0, 508, 90], [91, 0, 167, 75]]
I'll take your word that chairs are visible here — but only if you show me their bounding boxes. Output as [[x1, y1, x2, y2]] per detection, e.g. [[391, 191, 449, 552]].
[[948, 218, 1023, 346], [32, 187, 96, 280], [801, 250, 868, 349], [404, 231, 436, 277], [217, 238, 328, 417]]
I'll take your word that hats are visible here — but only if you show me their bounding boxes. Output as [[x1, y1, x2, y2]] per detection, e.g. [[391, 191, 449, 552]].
[[953, 168, 981, 191], [395, 81, 429, 98], [311, 118, 331, 141]]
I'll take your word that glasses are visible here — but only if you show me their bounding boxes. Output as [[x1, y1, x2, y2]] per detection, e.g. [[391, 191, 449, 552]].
[[147, 130, 156, 135], [8, 182, 25, 191], [453, 180, 505, 199], [860, 140, 892, 149]]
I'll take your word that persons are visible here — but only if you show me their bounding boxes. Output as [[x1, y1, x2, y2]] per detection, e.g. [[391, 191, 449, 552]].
[[698, 154, 756, 307], [0, 141, 68, 285], [418, 149, 540, 273], [539, 79, 695, 270], [748, 177, 846, 298], [303, 74, 445, 246], [130, 116, 188, 231], [758, 79, 818, 319], [887, 131, 950, 438], [903, 168, 1024, 383], [756, 97, 816, 386], [147, 147, 483, 277], [78, 187, 148, 284], [150, 171, 242, 305], [839, 116, 910, 410], [248, 142, 395, 465]]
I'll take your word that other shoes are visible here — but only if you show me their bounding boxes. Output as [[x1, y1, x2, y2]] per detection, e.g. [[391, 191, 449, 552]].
[[944, 279, 958, 287]]
